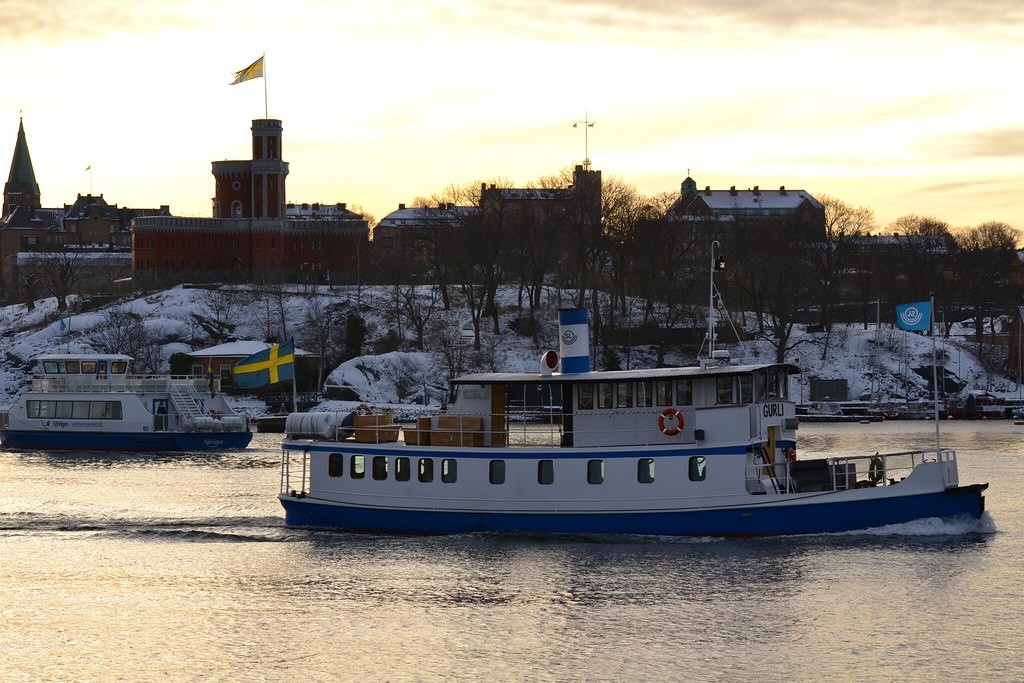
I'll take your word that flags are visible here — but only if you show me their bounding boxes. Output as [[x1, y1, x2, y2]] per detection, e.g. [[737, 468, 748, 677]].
[[229, 55, 263, 85], [60, 319, 66, 332], [232, 338, 295, 388], [205, 358, 215, 399], [896, 301, 933, 331]]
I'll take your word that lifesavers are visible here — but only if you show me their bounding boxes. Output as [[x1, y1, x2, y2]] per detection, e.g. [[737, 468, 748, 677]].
[[58, 378, 66, 392], [206, 409, 217, 420], [869, 459, 883, 482], [48, 378, 56, 391], [358, 404, 370, 415], [658, 408, 684, 436], [786, 447, 795, 465]]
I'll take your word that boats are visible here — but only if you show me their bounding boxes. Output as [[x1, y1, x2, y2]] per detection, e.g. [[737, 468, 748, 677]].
[[0, 312, 254, 451], [278, 243, 989, 545]]
